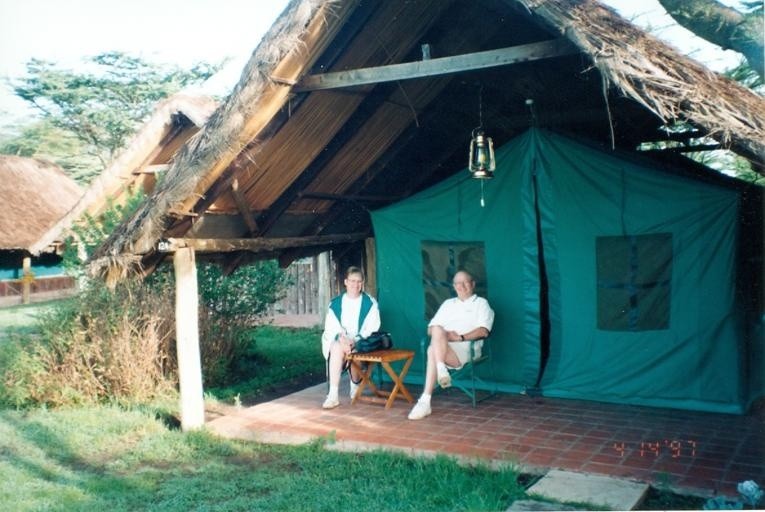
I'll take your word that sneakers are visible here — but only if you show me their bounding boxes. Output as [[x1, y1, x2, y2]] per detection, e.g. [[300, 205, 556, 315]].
[[436, 361, 452, 389], [349, 376, 364, 400], [407, 393, 432, 421], [322, 393, 340, 409]]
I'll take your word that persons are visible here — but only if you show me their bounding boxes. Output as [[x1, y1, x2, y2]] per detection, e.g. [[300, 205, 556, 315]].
[[407, 271, 495, 420]]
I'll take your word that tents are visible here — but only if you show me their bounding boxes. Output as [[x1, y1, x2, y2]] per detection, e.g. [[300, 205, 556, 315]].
[[321, 266, 381, 409], [365, 123, 765, 416]]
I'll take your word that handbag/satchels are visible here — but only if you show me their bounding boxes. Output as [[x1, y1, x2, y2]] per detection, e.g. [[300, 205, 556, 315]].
[[354, 330, 393, 353]]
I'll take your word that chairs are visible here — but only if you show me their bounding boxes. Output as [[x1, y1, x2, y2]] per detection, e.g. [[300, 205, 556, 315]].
[[326, 351, 383, 395], [419, 332, 497, 407]]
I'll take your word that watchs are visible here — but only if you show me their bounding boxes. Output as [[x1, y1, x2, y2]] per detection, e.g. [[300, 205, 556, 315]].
[[460, 334, 465, 341]]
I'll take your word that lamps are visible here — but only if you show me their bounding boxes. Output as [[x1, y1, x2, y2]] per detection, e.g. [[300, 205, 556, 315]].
[[468, 82, 496, 206]]
